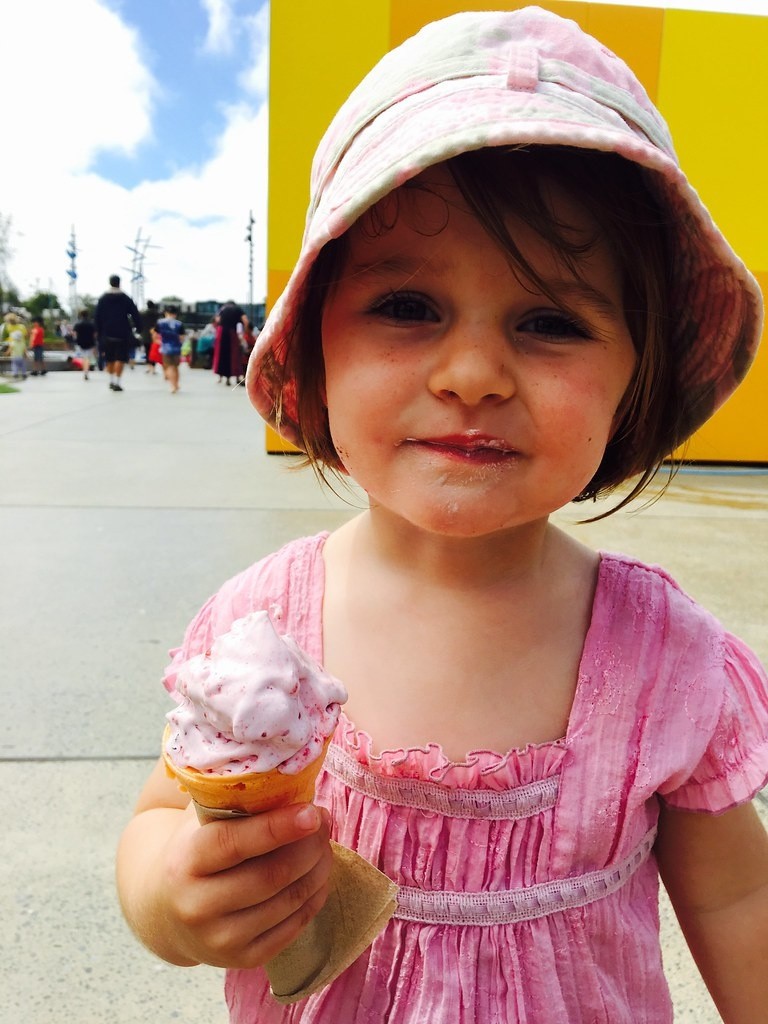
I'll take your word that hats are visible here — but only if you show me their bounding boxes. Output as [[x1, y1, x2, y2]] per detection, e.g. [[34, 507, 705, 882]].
[[10, 331, 22, 340], [243, 4, 764, 479]]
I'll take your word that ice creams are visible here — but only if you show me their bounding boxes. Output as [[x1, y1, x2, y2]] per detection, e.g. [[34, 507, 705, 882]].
[[162, 612, 348, 814]]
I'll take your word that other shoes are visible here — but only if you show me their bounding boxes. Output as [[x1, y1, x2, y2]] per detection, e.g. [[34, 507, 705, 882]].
[[110, 382, 123, 391], [22, 374, 27, 378], [41, 369, 47, 375], [13, 374, 18, 378], [30, 371, 38, 375]]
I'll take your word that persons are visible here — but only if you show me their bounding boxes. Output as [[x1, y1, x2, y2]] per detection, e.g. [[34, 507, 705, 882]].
[[1, 274, 260, 392], [116, 5, 768, 1024]]
